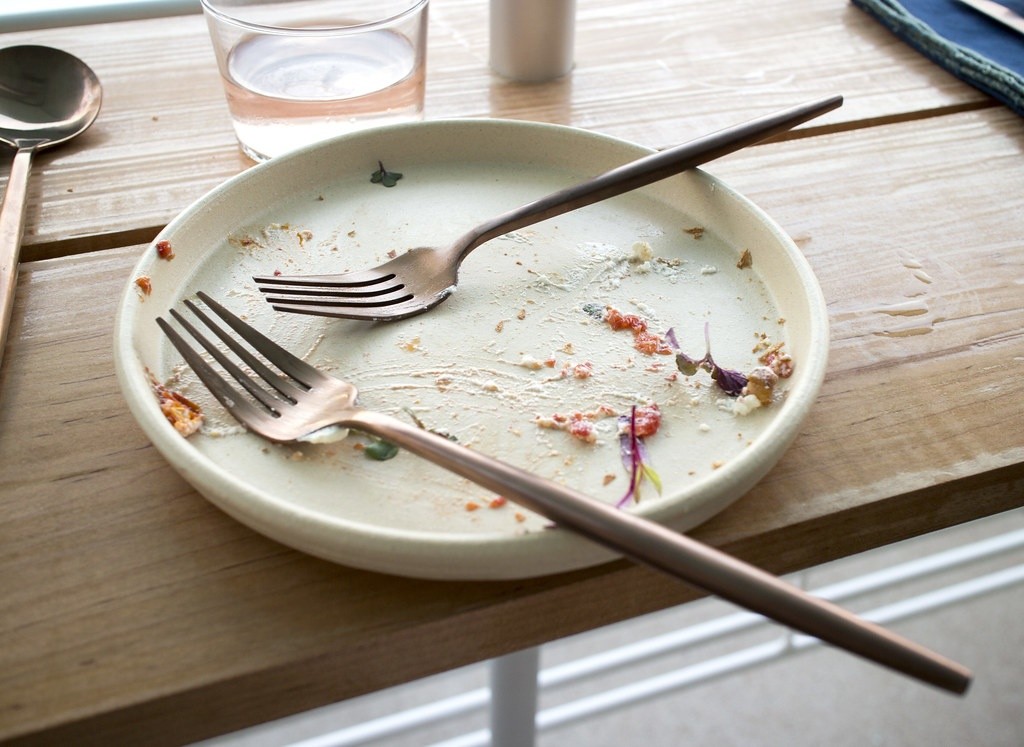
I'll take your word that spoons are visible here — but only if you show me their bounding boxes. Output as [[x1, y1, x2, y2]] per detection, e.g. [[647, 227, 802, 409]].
[[0, 45, 102, 363]]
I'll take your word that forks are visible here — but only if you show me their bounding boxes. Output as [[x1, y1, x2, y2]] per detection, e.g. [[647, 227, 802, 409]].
[[157, 292, 973, 698], [252, 94, 844, 321]]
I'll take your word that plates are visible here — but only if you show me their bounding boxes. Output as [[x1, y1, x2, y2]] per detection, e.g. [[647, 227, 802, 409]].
[[115, 118, 829, 581]]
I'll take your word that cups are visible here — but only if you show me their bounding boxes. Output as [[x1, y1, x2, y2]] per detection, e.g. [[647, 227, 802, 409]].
[[490, 0, 577, 82], [200, 0, 428, 165]]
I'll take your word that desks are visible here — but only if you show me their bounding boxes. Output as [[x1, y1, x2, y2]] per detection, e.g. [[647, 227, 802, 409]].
[[0, 0, 1024, 747]]
